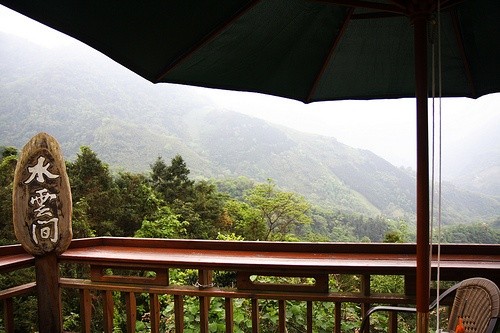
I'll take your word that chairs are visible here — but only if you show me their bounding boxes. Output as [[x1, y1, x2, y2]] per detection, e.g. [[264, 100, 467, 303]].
[[363, 278, 500, 333]]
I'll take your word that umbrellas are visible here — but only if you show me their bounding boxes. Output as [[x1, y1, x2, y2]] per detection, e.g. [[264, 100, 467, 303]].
[[0, 0, 500, 333]]
